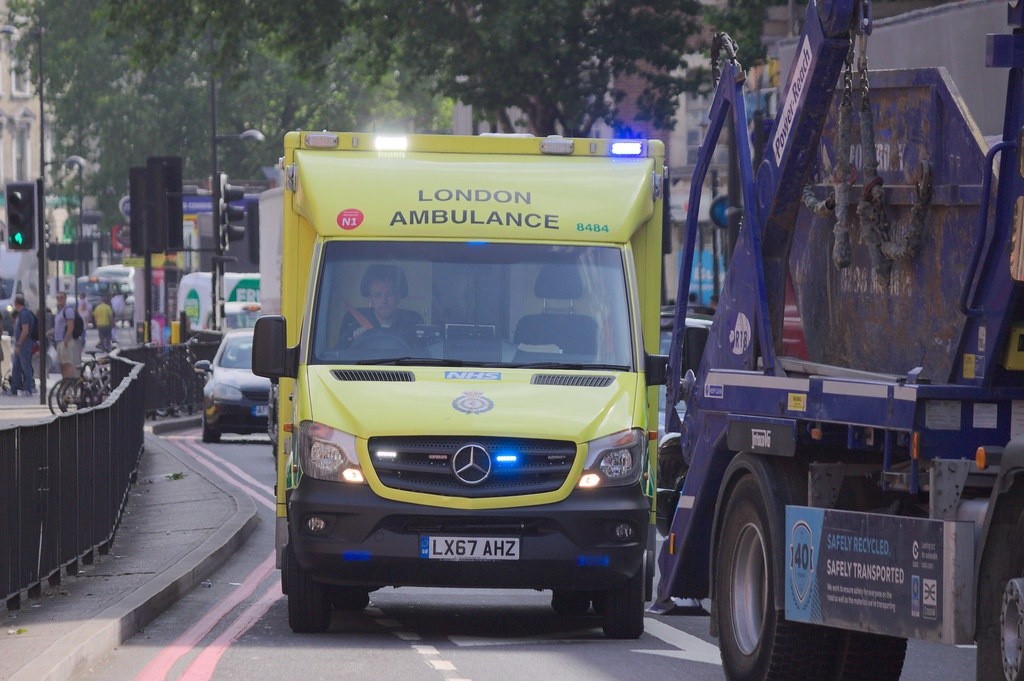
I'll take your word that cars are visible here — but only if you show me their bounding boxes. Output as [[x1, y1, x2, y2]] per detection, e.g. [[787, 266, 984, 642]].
[[65, 264, 135, 327], [194, 327, 277, 443]]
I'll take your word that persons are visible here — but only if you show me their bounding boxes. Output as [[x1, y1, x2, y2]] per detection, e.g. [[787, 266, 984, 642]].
[[340, 278, 437, 358], [0, 291, 135, 397]]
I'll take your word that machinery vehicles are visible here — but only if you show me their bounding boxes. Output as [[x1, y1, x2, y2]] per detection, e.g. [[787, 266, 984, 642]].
[[659, 0, 1024, 681]]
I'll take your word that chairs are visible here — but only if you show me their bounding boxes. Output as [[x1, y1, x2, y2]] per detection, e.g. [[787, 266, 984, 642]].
[[513, 267, 597, 355], [343, 265, 426, 340]]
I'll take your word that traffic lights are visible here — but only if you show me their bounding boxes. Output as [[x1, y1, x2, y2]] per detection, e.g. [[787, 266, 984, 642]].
[[217, 171, 246, 254], [4, 179, 36, 252]]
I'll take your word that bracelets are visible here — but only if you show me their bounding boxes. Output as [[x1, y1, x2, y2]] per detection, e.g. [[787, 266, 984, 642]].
[[15, 343, 20, 346]]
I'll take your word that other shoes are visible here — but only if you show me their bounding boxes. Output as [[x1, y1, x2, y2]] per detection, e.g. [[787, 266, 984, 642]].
[[10, 388, 22, 394], [17, 388, 31, 396]]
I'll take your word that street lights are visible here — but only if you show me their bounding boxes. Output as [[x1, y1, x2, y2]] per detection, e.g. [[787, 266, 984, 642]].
[[206, 65, 266, 326]]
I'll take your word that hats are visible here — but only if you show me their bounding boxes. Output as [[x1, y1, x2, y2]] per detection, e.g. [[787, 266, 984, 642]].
[[12, 293, 25, 305]]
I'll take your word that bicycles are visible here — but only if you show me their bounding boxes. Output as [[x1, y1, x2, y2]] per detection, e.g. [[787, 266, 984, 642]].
[[47, 349, 117, 419]]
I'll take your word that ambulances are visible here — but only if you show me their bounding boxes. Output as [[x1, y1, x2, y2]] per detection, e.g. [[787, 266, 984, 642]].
[[249, 128, 716, 640]]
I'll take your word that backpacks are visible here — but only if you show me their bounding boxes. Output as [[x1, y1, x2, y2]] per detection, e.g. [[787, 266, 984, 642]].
[[19, 309, 39, 339], [57, 306, 83, 339]]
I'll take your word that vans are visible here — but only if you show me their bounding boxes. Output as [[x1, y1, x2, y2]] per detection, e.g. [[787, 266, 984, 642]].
[[177, 270, 263, 343], [0, 241, 42, 326]]
